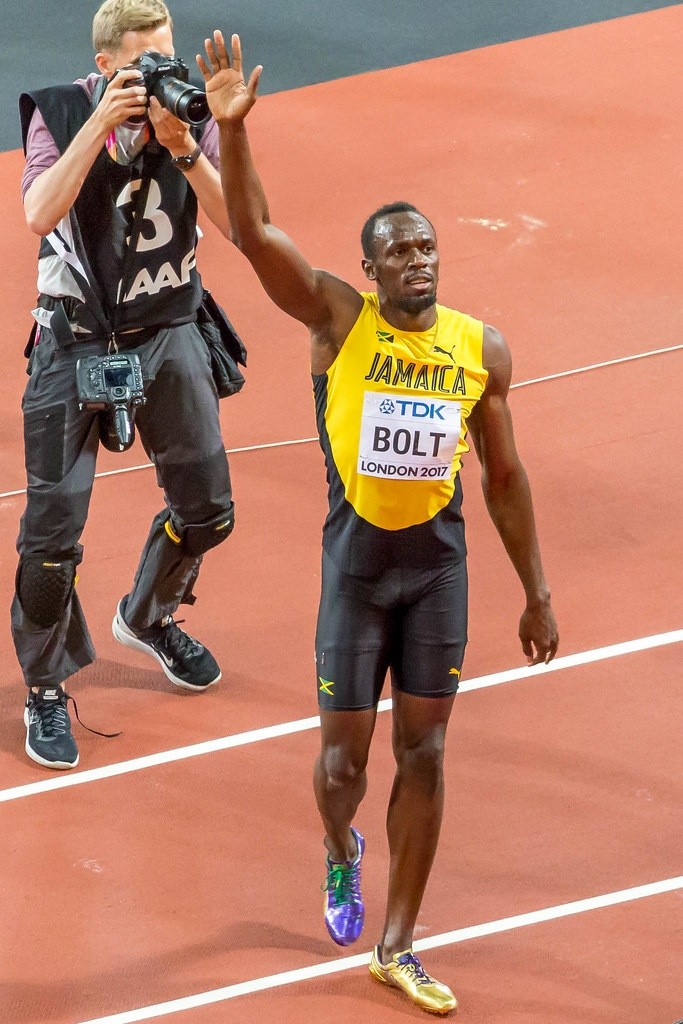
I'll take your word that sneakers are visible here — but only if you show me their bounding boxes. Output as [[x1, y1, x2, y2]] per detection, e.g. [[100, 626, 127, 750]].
[[23, 687, 80, 768], [322, 827, 367, 947], [369, 943, 458, 1015], [111, 594, 223, 691]]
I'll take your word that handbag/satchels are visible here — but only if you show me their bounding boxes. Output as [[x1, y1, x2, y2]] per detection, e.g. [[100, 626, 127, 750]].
[[196, 286, 248, 398]]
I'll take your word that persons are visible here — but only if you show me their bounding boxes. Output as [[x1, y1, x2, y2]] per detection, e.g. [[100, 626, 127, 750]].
[[197, 28, 559, 1014], [7, 0, 250, 771]]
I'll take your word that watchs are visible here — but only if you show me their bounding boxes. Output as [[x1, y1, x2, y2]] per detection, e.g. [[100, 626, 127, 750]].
[[170, 145, 202, 172]]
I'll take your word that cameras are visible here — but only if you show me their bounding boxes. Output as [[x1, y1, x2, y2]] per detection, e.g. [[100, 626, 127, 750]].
[[111, 52, 214, 126], [74, 353, 147, 453]]
